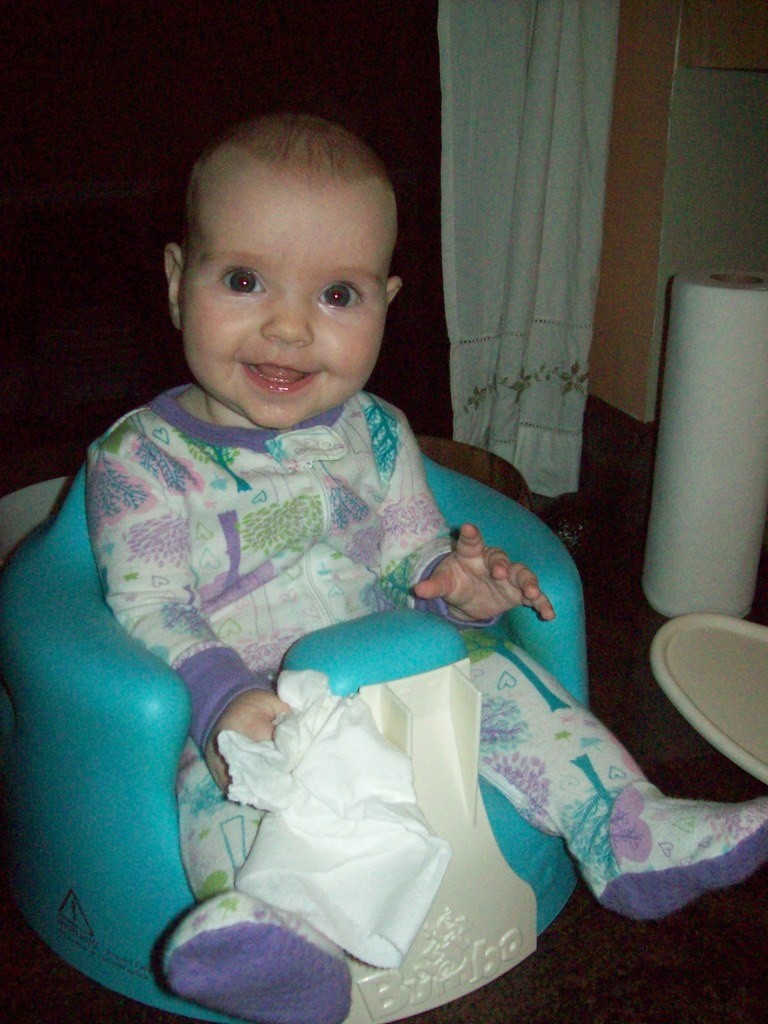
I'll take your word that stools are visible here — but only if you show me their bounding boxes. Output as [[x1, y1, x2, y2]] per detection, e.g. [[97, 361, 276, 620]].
[[0, 454, 590, 1024]]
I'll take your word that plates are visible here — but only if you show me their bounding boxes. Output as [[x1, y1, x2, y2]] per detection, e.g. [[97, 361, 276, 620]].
[[651, 614, 767, 785]]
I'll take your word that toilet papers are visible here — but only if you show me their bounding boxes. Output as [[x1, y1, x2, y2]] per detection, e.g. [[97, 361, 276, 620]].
[[640, 270, 768, 619]]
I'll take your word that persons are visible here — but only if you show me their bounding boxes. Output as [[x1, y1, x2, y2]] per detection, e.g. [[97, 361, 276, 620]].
[[85, 114, 768, 1024]]
